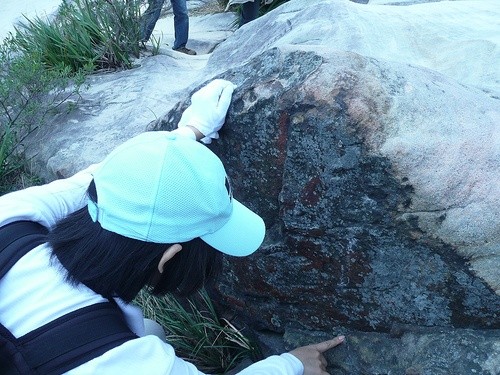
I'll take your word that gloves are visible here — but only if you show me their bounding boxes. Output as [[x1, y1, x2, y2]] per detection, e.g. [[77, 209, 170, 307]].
[[178, 78, 237, 144]]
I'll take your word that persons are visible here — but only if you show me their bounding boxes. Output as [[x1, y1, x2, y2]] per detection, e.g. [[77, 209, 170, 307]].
[[138, 0, 274, 55], [0, 78, 346, 375]]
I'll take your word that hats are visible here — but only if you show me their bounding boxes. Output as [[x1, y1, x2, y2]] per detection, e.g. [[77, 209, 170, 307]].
[[87, 131, 265, 257]]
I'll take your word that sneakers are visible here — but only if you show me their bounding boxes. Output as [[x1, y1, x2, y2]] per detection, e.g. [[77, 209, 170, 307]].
[[175, 47, 196, 55]]
